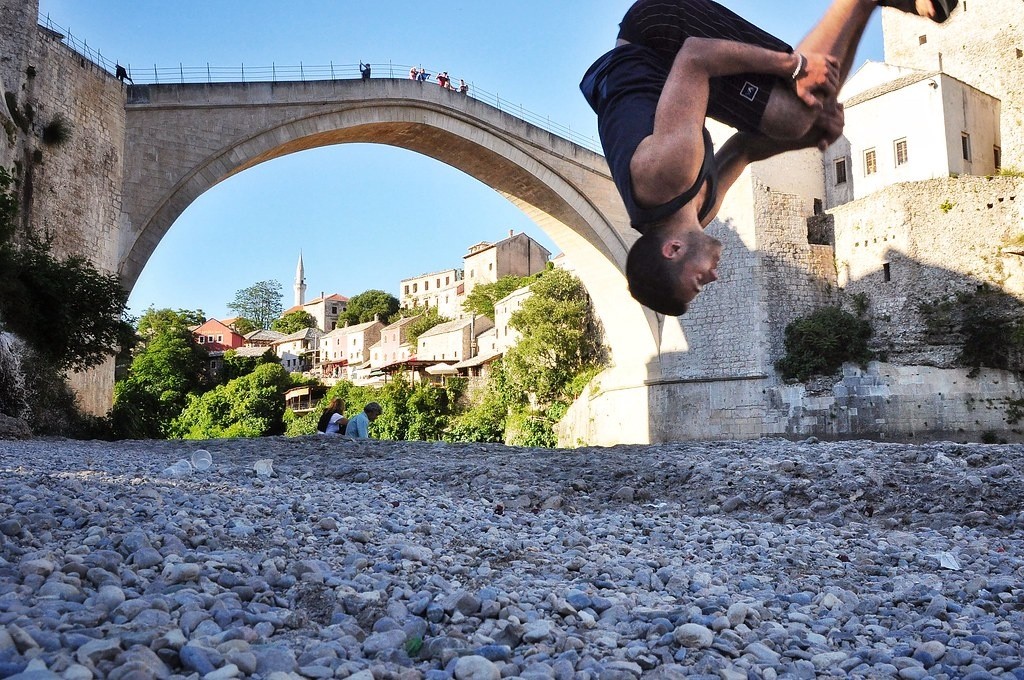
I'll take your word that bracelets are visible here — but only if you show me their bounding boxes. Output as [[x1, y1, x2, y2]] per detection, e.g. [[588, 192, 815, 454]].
[[784, 52, 802, 82]]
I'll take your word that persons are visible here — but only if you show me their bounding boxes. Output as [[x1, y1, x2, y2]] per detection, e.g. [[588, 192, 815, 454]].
[[360, 63, 371, 78], [317, 400, 382, 438], [437, 71, 468, 94], [409, 68, 428, 81], [115, 65, 134, 85], [579, 0, 958, 316]]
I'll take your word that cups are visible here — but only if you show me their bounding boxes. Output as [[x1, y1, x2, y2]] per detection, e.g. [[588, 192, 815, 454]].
[[191, 450, 213, 470], [163, 458, 192, 478]]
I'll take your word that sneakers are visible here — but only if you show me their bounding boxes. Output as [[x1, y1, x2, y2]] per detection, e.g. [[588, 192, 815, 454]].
[[873, 0, 962, 26]]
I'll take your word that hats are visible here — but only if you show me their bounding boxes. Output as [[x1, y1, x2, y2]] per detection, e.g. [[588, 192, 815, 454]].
[[443, 71, 448, 73]]
